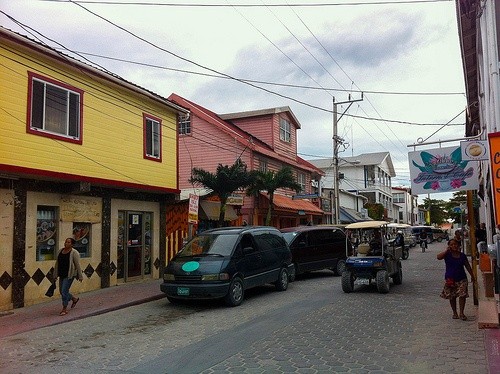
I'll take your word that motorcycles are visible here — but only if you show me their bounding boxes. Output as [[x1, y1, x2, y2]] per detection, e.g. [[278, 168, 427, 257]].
[[341, 221, 411, 294]]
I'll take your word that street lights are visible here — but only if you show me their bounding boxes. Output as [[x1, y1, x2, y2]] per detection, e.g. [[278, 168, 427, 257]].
[[335, 159, 360, 224]]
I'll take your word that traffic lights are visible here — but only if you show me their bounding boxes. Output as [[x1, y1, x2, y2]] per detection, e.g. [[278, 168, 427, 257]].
[[453, 213, 459, 224]]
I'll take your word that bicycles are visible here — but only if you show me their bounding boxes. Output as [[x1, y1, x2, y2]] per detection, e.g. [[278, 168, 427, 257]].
[[420, 238, 426, 253]]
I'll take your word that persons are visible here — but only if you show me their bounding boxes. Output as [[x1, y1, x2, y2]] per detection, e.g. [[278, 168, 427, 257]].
[[481, 223, 486, 230], [374, 232, 388, 256], [463, 227, 467, 241], [475, 223, 485, 258], [391, 229, 396, 235], [455, 230, 462, 252], [396, 232, 405, 259], [54, 238, 84, 316], [436, 239, 477, 319], [397, 228, 404, 235], [477, 234, 487, 255], [419, 229, 427, 249]]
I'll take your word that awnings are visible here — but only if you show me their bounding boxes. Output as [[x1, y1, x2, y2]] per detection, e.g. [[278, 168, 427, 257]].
[[200, 202, 239, 222]]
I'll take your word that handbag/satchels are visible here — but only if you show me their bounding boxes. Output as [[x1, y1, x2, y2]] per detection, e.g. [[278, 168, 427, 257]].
[[445, 276, 454, 287]]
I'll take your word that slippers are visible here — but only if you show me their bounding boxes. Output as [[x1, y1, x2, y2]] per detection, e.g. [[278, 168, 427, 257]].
[[71, 298, 79, 309], [59, 311, 68, 316]]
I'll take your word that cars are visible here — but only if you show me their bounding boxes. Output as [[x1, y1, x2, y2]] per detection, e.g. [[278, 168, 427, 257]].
[[278, 226, 352, 276]]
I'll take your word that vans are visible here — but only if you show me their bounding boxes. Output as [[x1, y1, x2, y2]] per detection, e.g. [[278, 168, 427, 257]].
[[397, 226, 445, 244], [159, 226, 295, 307]]
